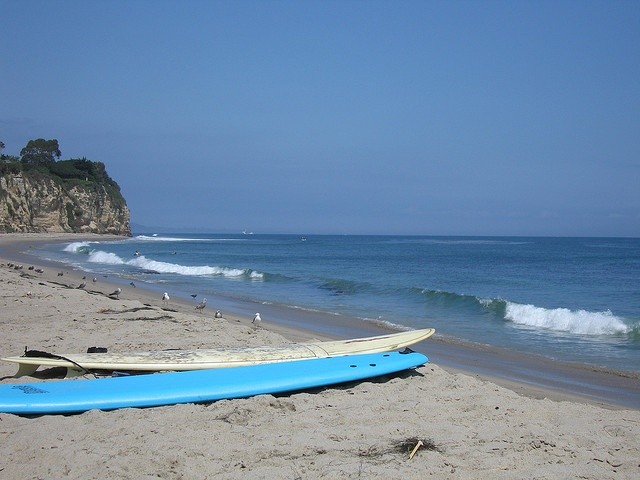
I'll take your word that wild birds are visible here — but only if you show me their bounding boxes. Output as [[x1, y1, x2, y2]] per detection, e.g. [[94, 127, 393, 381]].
[[75, 282, 87, 290], [215, 310, 222, 318], [161, 293, 170, 305], [251, 312, 262, 327], [194, 298, 208, 313], [93, 277, 98, 282], [109, 288, 122, 299]]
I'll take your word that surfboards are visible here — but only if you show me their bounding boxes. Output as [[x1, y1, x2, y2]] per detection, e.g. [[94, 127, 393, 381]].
[[0, 353, 428, 415], [0, 328, 435, 371]]
